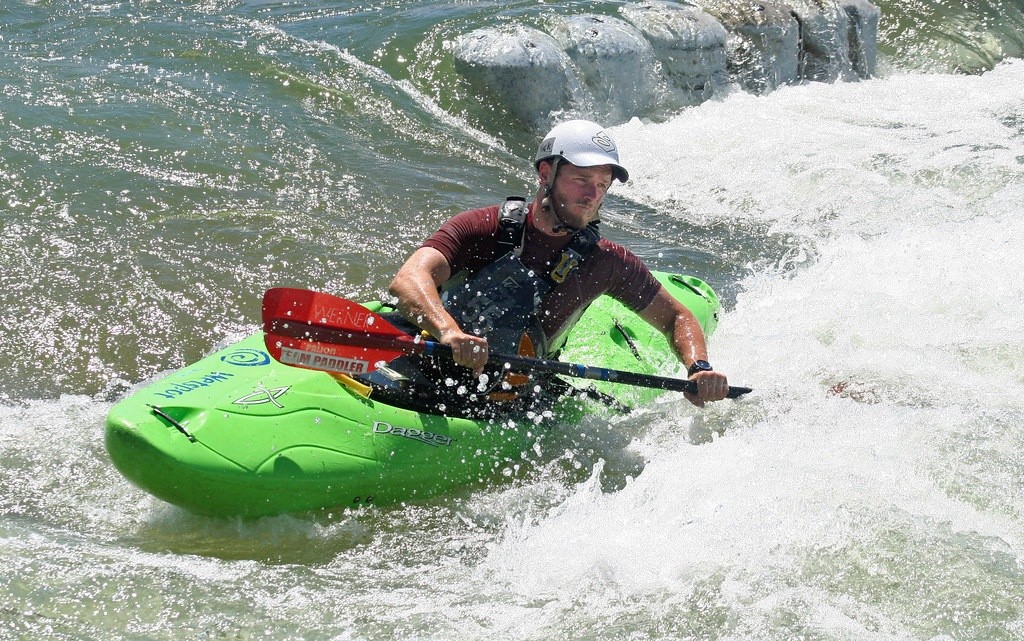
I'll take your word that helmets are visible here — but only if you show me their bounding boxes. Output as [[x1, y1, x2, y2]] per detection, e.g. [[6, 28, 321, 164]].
[[534, 119, 628, 183]]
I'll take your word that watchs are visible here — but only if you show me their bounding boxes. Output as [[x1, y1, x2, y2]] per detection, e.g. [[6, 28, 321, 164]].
[[688, 360, 713, 378]]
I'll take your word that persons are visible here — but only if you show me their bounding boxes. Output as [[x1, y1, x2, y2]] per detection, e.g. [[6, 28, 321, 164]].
[[350, 120, 729, 425]]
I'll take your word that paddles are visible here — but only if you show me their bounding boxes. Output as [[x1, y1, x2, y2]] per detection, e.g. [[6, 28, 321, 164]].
[[261, 286, 754, 400]]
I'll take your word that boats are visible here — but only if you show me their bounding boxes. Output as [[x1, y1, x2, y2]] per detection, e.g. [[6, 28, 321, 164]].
[[103, 273, 721, 520]]
